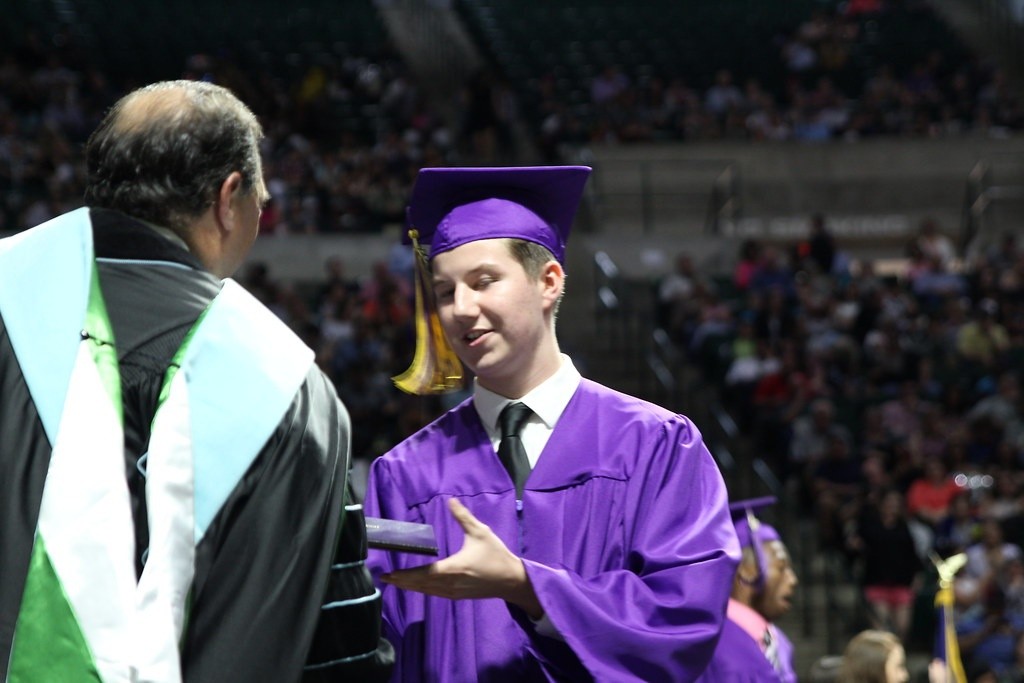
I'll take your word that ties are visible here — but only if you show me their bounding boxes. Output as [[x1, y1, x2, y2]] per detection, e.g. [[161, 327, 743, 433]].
[[497, 403, 534, 500]]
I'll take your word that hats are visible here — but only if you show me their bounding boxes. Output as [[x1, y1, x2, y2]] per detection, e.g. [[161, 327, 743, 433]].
[[728, 496, 785, 589], [390, 166, 593, 396]]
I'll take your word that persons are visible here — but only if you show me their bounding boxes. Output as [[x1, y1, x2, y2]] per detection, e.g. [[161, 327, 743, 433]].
[[365, 165, 742, 683], [0, 0, 1024, 683]]
